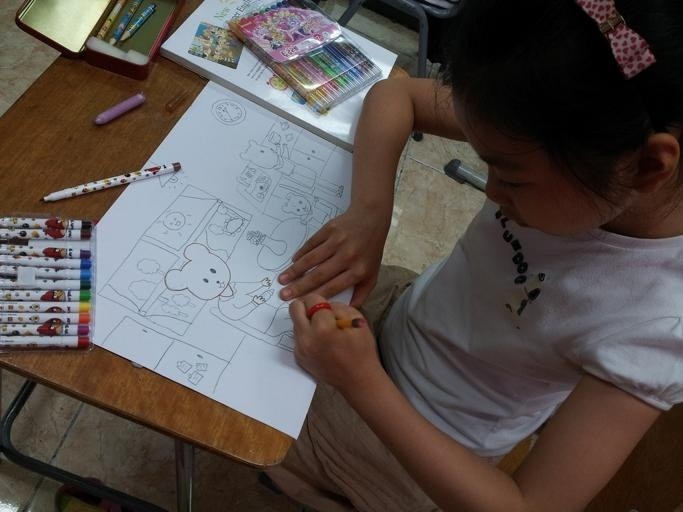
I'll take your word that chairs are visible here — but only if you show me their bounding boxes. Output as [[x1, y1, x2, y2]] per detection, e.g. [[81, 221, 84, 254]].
[[336, 0, 454, 141]]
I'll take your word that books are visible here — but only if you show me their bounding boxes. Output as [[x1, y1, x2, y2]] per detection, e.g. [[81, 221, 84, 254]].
[[158, 0, 399, 154]]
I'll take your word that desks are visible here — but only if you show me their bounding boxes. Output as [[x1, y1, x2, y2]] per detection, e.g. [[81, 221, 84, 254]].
[[0, 0, 411, 512]]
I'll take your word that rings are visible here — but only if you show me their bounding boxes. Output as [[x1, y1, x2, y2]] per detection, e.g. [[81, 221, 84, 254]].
[[307, 303, 331, 320]]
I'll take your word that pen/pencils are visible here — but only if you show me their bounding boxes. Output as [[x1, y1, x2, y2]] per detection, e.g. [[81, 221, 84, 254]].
[[109, 0, 141, 46], [95, 92, 146, 125], [335, 317, 367, 329], [39, 162, 190, 203], [120, 3, 157, 42], [0, 217, 92, 347], [228, 0, 383, 115], [98, 0, 126, 41]]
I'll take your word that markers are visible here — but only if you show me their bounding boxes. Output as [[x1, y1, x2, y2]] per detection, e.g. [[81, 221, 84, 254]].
[[444, 155, 487, 191]]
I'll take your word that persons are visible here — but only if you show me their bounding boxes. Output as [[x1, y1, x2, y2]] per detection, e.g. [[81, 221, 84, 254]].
[[263, 0, 683, 512]]
